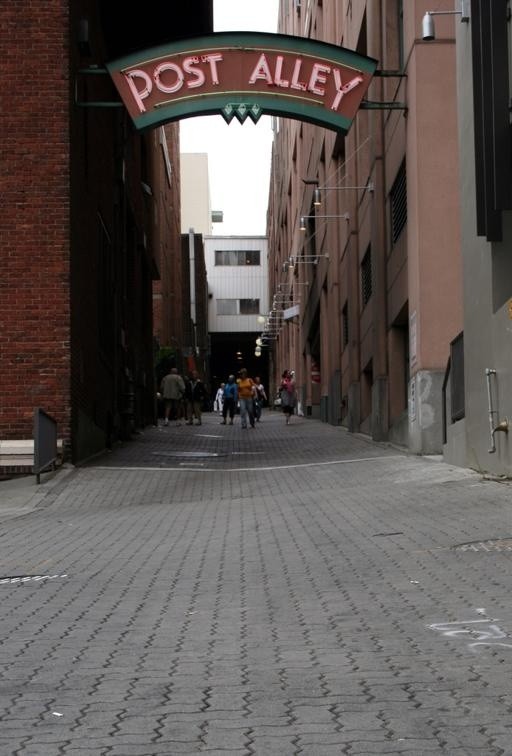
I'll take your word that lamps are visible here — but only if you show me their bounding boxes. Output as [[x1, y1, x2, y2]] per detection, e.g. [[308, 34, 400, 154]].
[[422, 8, 462, 42], [255, 135, 374, 357]]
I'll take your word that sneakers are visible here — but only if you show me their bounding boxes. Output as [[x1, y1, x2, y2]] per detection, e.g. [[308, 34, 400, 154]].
[[220, 422, 225, 424], [229, 422, 233, 425], [164, 422, 167, 425], [185, 421, 193, 425], [195, 423, 201, 425], [176, 422, 181, 427]]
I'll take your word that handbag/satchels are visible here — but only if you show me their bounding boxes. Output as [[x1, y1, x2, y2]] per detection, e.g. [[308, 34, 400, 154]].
[[214, 401, 217, 413]]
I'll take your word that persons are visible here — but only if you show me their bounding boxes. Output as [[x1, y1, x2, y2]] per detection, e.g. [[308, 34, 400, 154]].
[[251, 376, 268, 422], [185, 370, 205, 426], [160, 367, 186, 428], [235, 371, 241, 384], [278, 369, 296, 425], [219, 374, 238, 425], [238, 368, 257, 429], [215, 383, 226, 416]]
